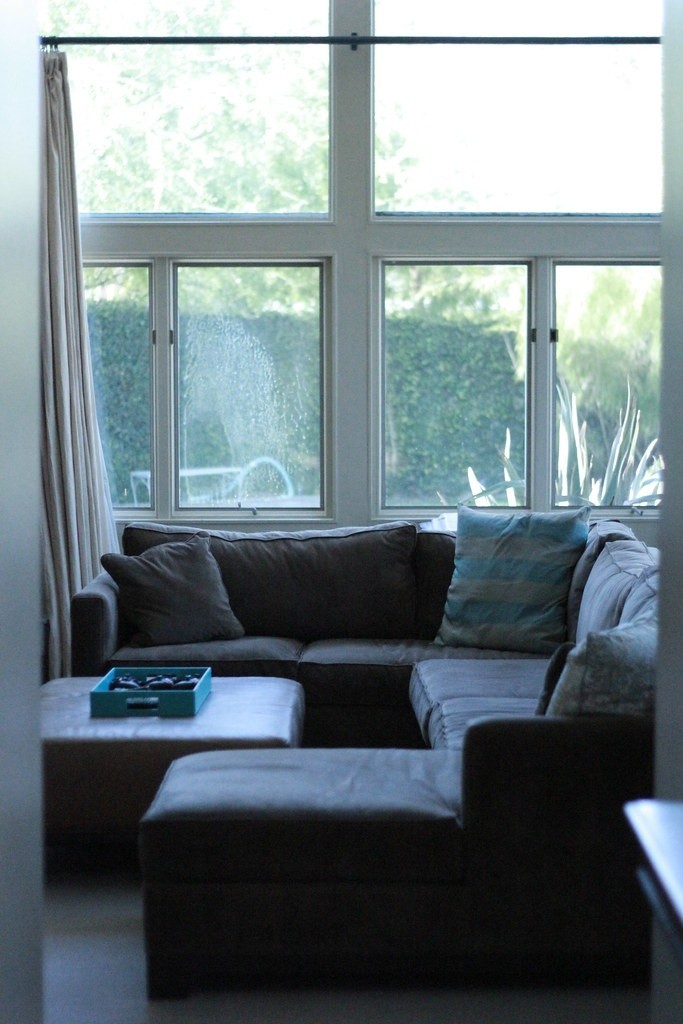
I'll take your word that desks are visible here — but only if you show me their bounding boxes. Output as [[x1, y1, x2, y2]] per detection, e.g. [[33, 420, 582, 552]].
[[619, 798, 683, 1024]]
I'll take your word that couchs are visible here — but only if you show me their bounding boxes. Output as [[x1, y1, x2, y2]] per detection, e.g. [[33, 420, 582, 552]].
[[69, 517, 657, 993]]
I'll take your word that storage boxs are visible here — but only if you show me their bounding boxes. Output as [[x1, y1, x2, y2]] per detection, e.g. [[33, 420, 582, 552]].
[[90, 667, 212, 717]]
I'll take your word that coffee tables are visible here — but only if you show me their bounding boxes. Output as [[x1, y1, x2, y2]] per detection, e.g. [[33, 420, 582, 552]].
[[41, 676, 303, 877]]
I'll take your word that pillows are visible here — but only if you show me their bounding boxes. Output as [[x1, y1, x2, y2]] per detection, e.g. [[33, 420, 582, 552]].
[[100, 531, 247, 649], [535, 641, 575, 715], [546, 610, 656, 718], [434, 503, 590, 656]]
